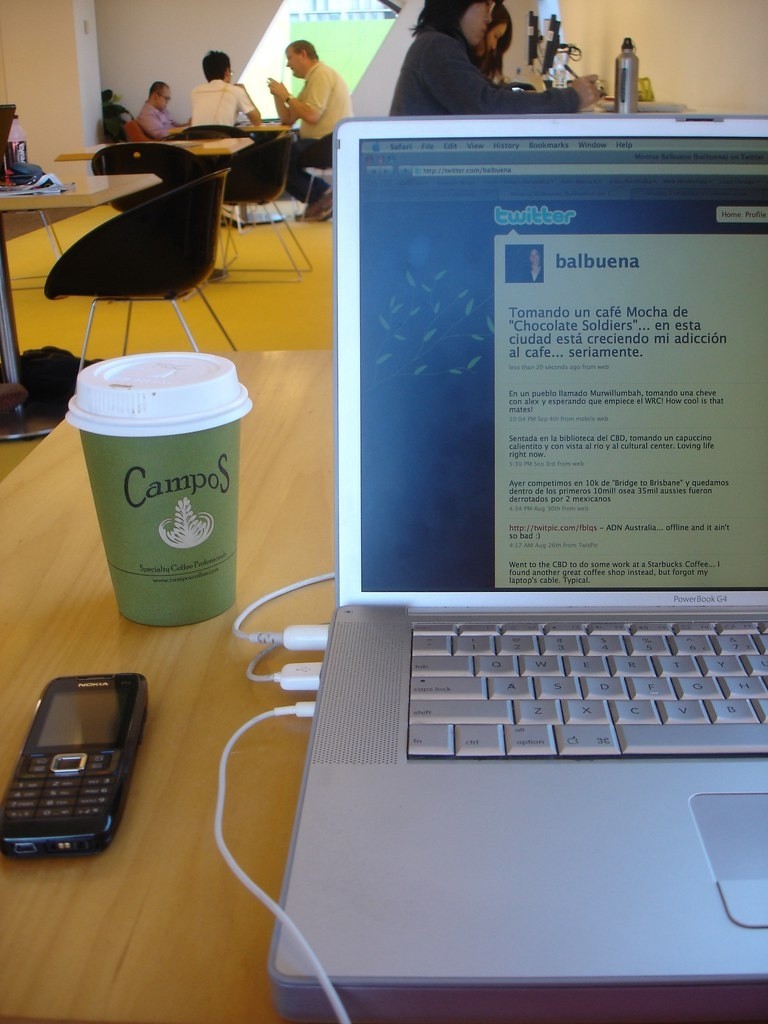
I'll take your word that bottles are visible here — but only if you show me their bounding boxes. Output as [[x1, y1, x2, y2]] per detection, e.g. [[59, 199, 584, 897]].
[[551, 45, 569, 89], [4, 115, 28, 171]]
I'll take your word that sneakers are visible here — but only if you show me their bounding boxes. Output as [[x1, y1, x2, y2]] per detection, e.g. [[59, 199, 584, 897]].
[[295, 188, 333, 222]]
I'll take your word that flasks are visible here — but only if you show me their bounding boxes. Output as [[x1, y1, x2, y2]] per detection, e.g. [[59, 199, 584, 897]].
[[614, 37, 639, 115]]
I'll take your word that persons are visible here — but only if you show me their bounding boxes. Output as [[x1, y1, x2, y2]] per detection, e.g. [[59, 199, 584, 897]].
[[267, 40, 355, 222], [136, 81, 191, 141], [191, 50, 261, 228], [467, 0, 512, 84], [387, 0, 601, 117], [522, 248, 544, 283]]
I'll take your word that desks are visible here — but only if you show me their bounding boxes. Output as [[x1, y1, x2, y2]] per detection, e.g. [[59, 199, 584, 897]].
[[0, 173, 163, 440], [52, 124, 295, 280], [0, 350, 339, 1023]]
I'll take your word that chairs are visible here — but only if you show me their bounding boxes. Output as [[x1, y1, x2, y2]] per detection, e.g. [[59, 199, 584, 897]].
[[92, 118, 334, 285], [43, 167, 239, 393]]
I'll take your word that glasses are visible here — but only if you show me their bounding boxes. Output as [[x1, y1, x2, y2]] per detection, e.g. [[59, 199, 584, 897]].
[[229, 69, 234, 76], [157, 92, 170, 102]]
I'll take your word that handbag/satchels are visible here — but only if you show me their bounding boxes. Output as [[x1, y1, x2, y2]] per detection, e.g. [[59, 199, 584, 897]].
[[638, 78, 654, 101]]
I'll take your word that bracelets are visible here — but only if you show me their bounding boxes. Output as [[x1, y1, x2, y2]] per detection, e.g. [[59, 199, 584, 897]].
[[286, 96, 294, 103]]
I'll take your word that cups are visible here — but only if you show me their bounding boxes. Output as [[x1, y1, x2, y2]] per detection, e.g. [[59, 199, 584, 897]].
[[65, 352, 256, 628]]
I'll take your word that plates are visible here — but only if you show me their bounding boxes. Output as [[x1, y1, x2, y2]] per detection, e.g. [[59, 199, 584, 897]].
[[596, 95, 687, 113]]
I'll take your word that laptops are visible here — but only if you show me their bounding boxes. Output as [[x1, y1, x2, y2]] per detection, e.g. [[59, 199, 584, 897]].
[[270, 112, 768, 987]]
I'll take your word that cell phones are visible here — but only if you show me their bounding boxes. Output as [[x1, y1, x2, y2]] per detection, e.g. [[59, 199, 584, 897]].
[[0, 673, 149, 859]]
